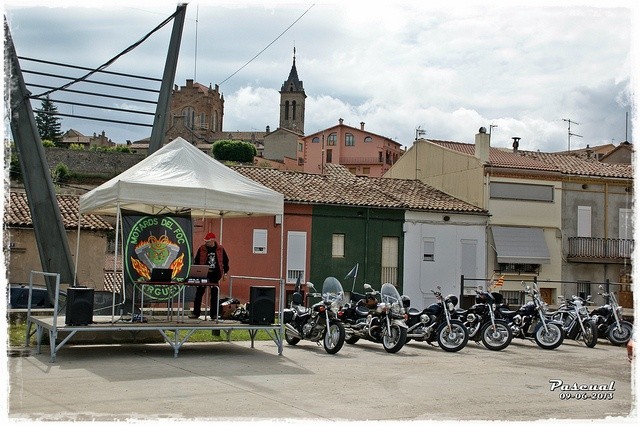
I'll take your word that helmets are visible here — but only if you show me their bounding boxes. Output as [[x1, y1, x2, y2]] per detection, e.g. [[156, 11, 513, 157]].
[[446, 293, 459, 306], [360, 293, 378, 309], [398, 294, 411, 308]]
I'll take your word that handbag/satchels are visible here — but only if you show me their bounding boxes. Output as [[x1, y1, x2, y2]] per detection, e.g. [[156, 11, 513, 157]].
[[221, 298, 243, 320], [231, 307, 249, 324]]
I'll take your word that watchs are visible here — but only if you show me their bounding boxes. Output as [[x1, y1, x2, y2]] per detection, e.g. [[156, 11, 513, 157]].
[[225, 273, 228, 275]]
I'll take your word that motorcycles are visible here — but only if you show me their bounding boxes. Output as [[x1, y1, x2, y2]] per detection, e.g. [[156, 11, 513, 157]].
[[588, 285, 634, 345], [283, 277, 352, 354], [542, 294, 597, 348], [455, 286, 512, 350], [498, 281, 565, 349], [400, 286, 469, 352], [339, 283, 408, 353]]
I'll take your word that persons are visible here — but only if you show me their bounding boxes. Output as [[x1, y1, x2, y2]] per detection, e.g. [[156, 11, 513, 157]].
[[188, 232, 229, 321]]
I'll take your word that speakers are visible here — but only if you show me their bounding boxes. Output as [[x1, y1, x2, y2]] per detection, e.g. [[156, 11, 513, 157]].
[[249, 286, 276, 325], [65, 287, 94, 325]]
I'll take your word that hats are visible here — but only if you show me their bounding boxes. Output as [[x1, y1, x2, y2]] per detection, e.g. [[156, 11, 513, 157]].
[[204, 232, 217, 241]]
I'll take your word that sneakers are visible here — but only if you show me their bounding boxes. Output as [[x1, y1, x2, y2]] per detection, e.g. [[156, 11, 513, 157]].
[[188, 312, 200, 318], [211, 315, 217, 320]]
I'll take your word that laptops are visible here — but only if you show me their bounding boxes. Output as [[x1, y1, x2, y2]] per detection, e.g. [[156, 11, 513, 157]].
[[147, 268, 172, 282], [185, 265, 209, 280]]
